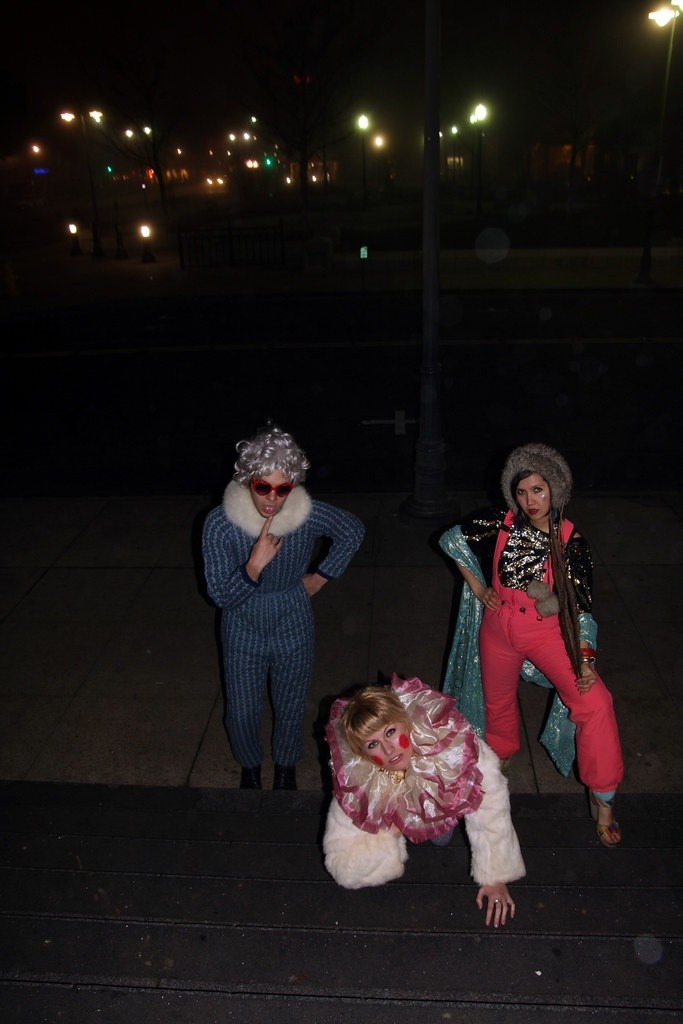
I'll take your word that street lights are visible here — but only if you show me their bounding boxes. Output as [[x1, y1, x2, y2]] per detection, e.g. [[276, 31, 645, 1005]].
[[354, 110, 373, 206], [632, 2, 683, 284], [227, 132, 252, 203], [449, 124, 461, 182], [468, 100, 491, 212], [126, 126, 153, 206], [60, 106, 104, 233]]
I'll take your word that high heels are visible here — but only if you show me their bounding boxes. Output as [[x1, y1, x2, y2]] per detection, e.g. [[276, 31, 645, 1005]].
[[588, 786, 624, 848]]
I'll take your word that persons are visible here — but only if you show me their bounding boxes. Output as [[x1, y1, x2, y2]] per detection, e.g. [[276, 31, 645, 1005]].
[[201, 428, 366, 791], [322, 673, 526, 928], [439, 445, 624, 847]]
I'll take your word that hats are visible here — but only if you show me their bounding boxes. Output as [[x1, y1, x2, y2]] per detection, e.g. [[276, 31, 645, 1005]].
[[500, 443, 573, 515]]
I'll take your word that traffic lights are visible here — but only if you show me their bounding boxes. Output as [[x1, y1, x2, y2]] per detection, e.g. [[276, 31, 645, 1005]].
[[106, 166, 112, 173], [264, 157, 272, 168]]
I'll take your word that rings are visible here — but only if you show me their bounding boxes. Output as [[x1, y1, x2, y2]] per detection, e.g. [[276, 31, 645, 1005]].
[[495, 899, 502, 903], [275, 538, 278, 543]]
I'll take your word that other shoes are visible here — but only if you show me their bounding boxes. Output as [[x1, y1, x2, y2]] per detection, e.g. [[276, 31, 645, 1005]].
[[238, 764, 262, 789], [273, 765, 298, 789]]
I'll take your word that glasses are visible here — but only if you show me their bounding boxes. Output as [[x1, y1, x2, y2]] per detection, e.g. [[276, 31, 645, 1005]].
[[252, 480, 297, 498]]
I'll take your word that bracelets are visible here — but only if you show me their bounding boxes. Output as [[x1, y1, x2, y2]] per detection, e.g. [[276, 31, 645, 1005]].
[[579, 648, 596, 662]]
